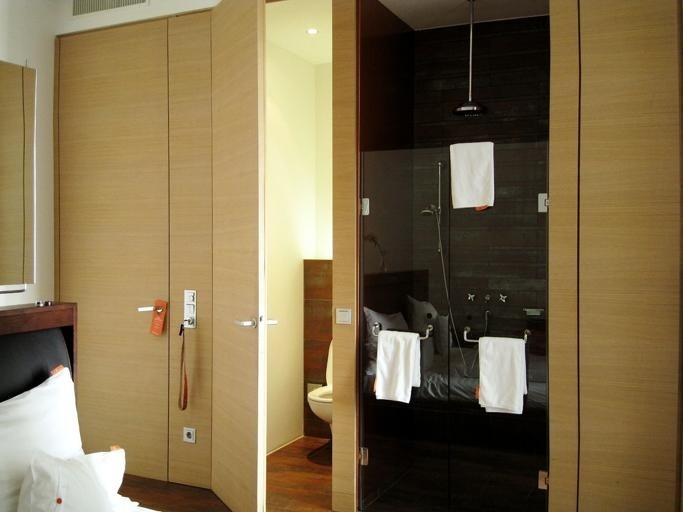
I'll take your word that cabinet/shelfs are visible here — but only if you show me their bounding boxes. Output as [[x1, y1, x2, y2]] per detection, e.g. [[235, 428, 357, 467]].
[[44, 1, 214, 491]]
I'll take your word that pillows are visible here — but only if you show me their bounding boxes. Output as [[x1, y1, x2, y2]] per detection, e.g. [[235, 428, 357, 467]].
[[364, 295, 453, 373], [0, 363, 127, 512]]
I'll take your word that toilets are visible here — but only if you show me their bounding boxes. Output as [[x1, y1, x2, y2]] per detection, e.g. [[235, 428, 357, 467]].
[[308, 339, 333, 434]]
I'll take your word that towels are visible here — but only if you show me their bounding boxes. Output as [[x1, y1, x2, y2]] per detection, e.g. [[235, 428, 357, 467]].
[[447, 141, 497, 211], [372, 330, 530, 418]]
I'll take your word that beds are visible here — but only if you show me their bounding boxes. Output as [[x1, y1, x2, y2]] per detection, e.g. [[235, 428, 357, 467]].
[[363, 269, 548, 406]]
